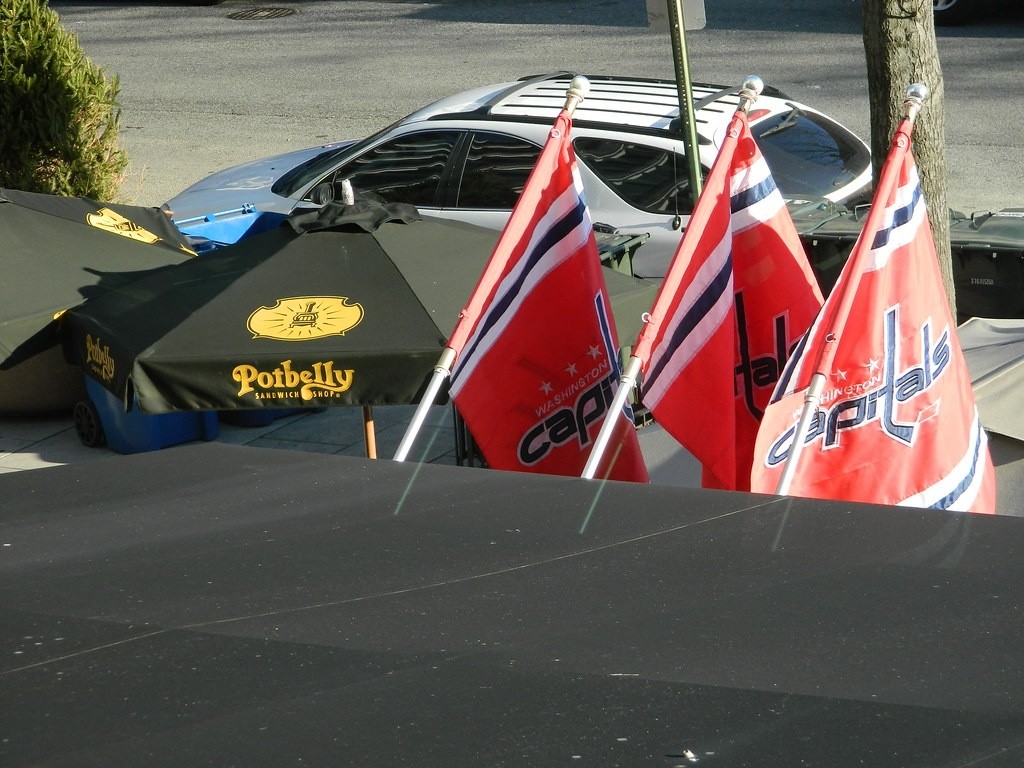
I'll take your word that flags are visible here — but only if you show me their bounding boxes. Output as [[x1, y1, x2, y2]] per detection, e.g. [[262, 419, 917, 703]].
[[445, 110, 650, 484], [750, 120, 995, 514], [628, 111, 826, 493]]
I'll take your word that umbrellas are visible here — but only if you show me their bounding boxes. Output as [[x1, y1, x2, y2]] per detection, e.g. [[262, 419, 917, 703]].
[[63, 180, 504, 459], [0, 187, 198, 374]]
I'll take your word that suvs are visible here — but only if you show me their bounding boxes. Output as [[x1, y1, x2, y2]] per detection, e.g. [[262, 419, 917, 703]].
[[160, 70, 874, 245]]
